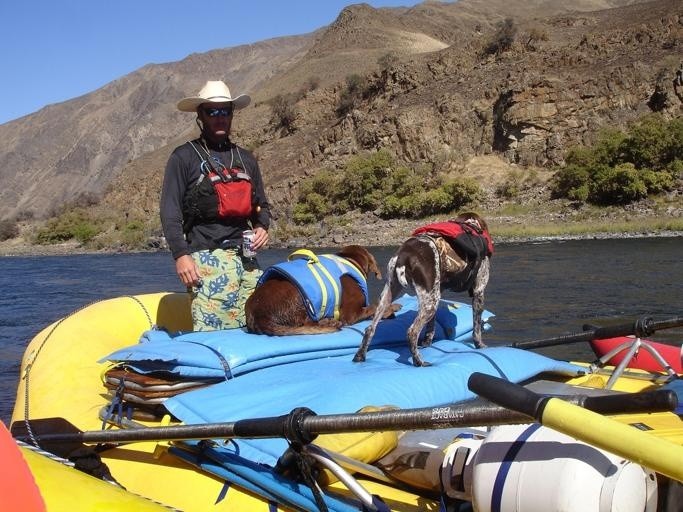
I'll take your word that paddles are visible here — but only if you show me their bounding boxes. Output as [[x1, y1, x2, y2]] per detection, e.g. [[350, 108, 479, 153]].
[[11, 389, 677, 460]]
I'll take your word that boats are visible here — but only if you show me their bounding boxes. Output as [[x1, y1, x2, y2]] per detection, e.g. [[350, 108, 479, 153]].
[[582, 323, 683, 378], [10, 292, 683, 512]]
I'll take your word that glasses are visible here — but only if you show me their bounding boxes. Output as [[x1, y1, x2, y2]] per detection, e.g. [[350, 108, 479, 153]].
[[200, 104, 232, 117]]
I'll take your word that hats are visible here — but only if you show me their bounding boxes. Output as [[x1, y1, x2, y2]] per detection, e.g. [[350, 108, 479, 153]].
[[177, 81, 251, 112]]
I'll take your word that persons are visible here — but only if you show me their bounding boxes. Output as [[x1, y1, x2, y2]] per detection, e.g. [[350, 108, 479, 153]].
[[160, 80, 274, 333]]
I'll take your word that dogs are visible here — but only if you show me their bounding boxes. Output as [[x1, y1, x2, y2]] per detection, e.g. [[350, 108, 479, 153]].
[[244, 244, 404, 337], [351, 211, 491, 368]]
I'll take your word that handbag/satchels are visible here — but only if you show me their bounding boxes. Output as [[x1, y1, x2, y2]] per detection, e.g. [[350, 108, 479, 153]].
[[191, 139, 256, 225]]
